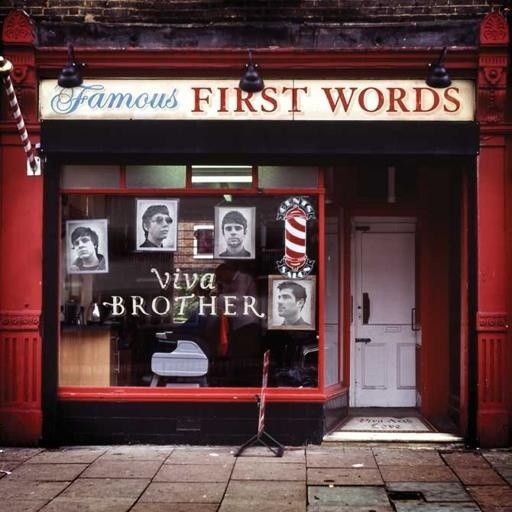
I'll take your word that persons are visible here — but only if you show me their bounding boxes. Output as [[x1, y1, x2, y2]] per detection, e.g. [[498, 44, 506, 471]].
[[276, 281, 311, 326], [218, 211, 250, 257], [139, 205, 173, 248], [69, 227, 105, 271]]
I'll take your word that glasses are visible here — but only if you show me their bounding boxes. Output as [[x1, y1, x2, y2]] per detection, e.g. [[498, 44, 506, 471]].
[[150, 216, 172, 224]]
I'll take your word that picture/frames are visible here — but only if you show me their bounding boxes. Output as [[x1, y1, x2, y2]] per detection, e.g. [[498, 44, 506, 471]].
[[214, 206, 256, 260], [65, 218, 109, 274], [135, 197, 180, 252], [267, 275, 316, 330]]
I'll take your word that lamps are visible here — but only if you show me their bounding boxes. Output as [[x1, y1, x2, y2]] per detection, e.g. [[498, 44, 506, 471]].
[[425, 48, 452, 88], [58, 44, 84, 87], [191, 175, 252, 183], [239, 52, 265, 92]]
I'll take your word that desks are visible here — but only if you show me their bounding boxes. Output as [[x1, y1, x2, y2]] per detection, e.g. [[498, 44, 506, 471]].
[[58, 319, 144, 386]]
